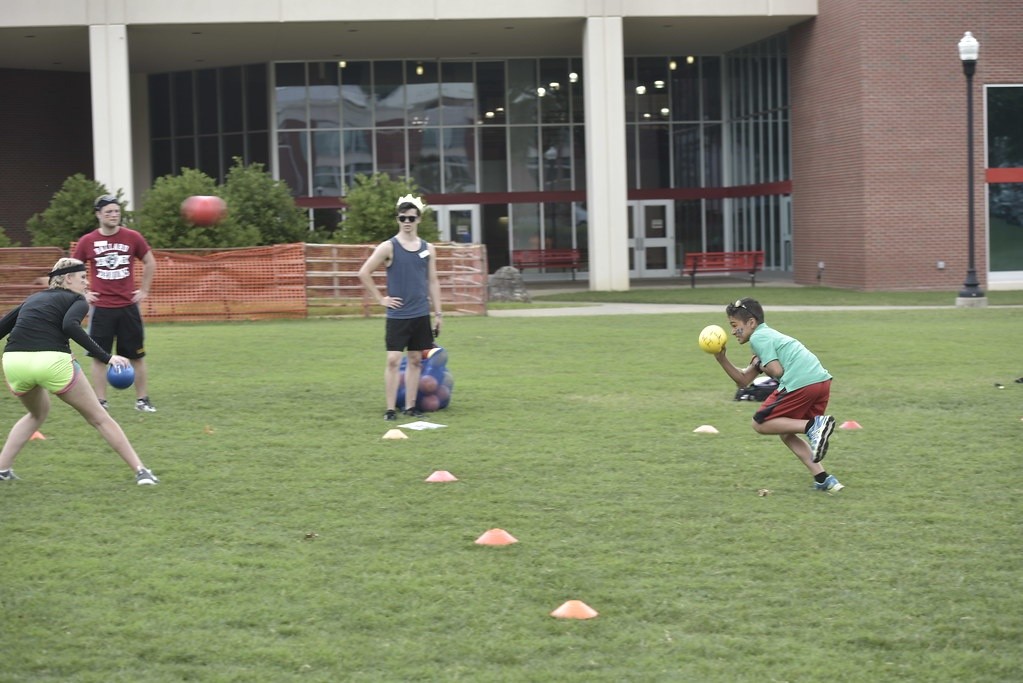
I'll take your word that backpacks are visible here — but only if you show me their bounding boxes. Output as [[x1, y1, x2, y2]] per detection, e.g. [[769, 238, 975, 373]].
[[734, 376, 780, 401]]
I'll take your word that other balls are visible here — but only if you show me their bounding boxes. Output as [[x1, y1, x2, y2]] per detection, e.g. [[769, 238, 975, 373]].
[[396, 346, 453, 413], [106, 362, 135, 390], [699, 325, 728, 354], [182, 196, 228, 227]]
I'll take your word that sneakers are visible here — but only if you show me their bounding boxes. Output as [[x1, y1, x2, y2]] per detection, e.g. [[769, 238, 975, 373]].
[[134, 394, 156, 413], [813, 474, 845, 492], [0, 468, 22, 481], [807, 415, 836, 463], [99, 399, 108, 409], [400, 407, 424, 418], [135, 466, 161, 486], [384, 409, 396, 421]]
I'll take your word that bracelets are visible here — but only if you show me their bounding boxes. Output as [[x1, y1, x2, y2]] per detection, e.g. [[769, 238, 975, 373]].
[[435, 311, 441, 317]]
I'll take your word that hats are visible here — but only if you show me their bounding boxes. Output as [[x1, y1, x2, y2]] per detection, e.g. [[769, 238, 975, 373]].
[[397, 194, 426, 215]]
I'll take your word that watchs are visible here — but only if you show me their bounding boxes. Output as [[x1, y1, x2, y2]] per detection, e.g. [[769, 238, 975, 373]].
[[756, 360, 763, 375]]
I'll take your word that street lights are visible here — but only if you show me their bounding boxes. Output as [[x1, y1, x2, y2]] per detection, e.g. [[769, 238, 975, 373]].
[[959, 31, 987, 298], [546, 146, 559, 251]]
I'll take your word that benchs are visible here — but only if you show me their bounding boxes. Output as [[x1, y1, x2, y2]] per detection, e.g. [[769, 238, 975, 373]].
[[681, 251, 764, 289], [512, 249, 580, 281]]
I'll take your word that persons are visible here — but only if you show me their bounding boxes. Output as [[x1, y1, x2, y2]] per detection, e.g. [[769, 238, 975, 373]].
[[359, 193, 442, 420], [713, 298, 845, 493], [71, 194, 157, 413], [0, 258, 160, 486]]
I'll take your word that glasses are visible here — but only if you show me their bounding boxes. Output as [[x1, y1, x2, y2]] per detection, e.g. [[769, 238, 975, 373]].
[[398, 215, 418, 222], [733, 299, 758, 320]]
